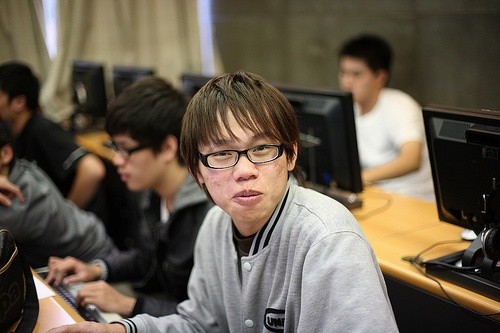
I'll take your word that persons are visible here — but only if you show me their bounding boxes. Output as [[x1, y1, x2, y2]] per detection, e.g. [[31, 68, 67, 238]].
[[335, 34, 435, 200], [0, 61, 216, 318], [47, 72, 399, 333]]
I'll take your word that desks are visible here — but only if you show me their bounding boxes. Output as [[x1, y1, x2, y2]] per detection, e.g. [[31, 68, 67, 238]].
[[13, 262, 85, 333], [62, 120, 500, 319]]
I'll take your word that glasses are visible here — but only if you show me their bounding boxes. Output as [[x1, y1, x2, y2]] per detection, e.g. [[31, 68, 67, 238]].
[[197, 144, 283, 170], [106, 139, 152, 157]]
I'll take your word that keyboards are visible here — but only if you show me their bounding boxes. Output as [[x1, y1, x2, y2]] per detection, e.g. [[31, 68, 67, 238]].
[[40, 271, 107, 326]]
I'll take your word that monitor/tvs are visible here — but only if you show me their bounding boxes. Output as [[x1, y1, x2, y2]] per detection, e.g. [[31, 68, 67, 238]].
[[275, 85, 363, 210], [71, 60, 217, 136], [423, 105, 500, 302]]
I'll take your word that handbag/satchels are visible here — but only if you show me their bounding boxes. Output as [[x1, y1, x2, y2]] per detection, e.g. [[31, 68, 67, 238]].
[[0, 228, 40, 333]]
